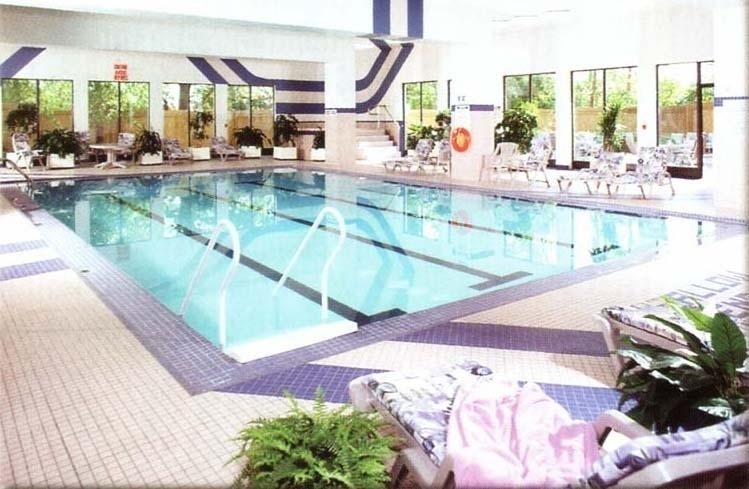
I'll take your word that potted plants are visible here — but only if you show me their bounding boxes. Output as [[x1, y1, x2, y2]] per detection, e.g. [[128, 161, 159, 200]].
[[32, 127, 92, 170], [129, 126, 164, 165], [232, 113, 325, 160]]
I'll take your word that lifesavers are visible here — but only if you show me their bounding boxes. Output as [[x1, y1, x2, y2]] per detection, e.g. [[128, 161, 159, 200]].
[[451, 128, 470, 152]]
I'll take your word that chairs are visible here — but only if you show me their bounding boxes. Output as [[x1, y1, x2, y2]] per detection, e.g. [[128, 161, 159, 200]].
[[480, 128, 712, 199], [10, 133, 45, 172], [165, 135, 241, 166], [346, 353, 749, 486], [383, 137, 451, 176]]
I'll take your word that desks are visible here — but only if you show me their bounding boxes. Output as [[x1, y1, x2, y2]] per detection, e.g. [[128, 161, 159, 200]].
[[89, 139, 128, 171]]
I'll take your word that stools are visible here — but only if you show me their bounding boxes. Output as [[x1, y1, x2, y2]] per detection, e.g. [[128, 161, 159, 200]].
[[594, 267, 747, 377]]
